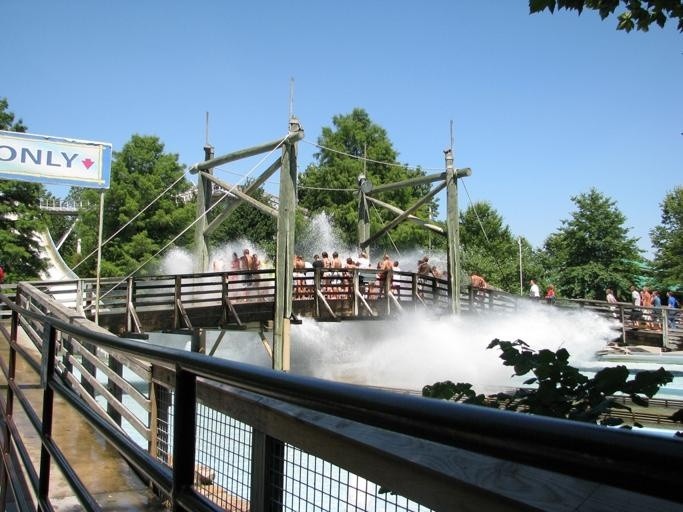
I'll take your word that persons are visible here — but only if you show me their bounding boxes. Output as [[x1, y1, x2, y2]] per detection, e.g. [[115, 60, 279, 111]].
[[630, 286, 679, 330], [529, 279, 555, 306], [467, 272, 486, 309], [227, 249, 261, 288], [606, 289, 618, 317], [417, 257, 448, 299], [293, 251, 401, 299]]
